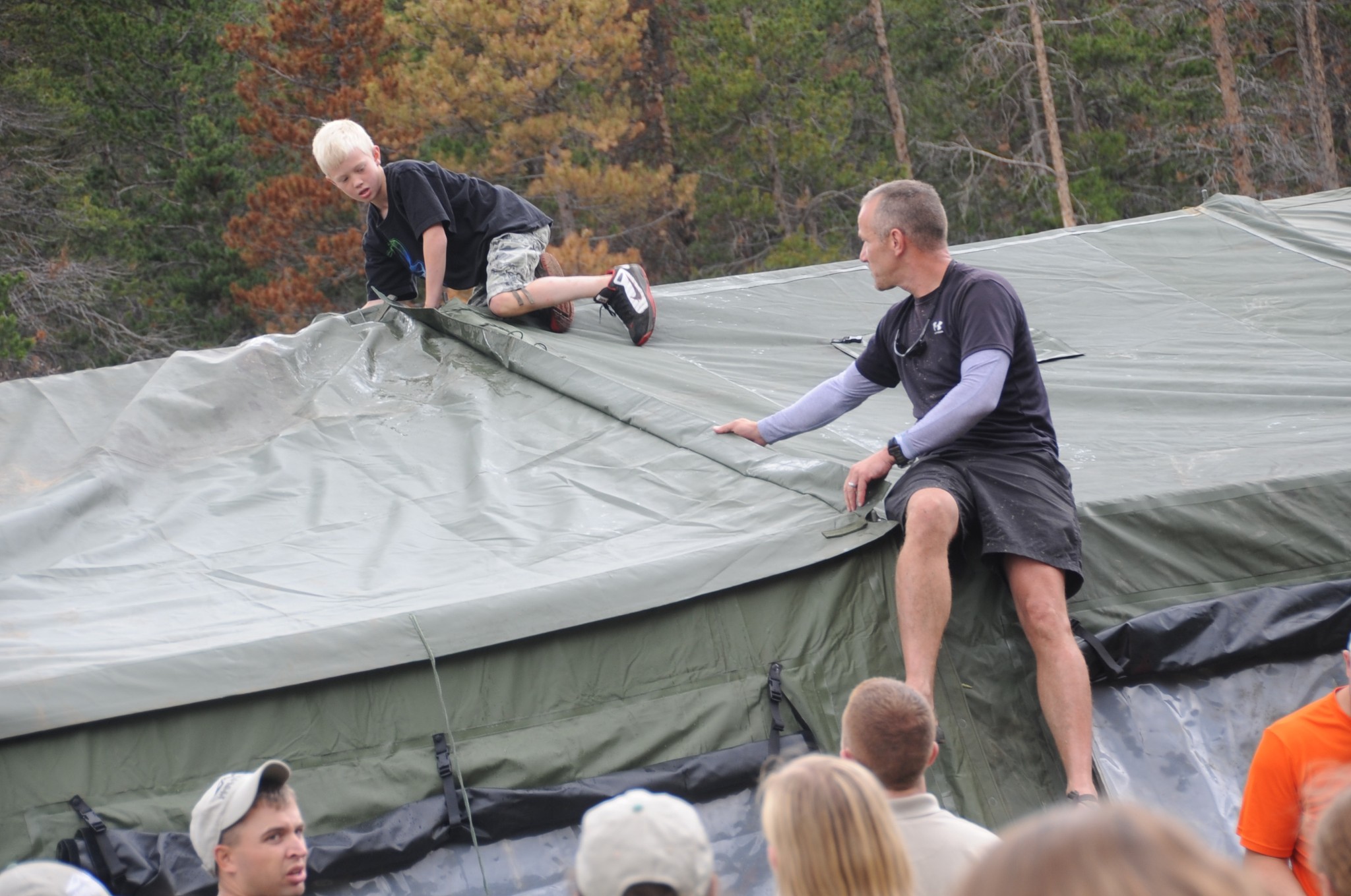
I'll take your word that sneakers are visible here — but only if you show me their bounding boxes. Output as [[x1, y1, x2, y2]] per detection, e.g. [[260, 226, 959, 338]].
[[536, 254, 574, 331], [593, 264, 656, 346]]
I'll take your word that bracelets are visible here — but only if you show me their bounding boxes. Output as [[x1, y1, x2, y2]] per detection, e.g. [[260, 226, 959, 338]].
[[887, 436, 916, 469]]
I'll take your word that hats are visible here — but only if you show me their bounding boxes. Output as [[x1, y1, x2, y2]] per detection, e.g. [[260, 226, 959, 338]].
[[574, 788, 715, 895], [184, 759, 291, 874]]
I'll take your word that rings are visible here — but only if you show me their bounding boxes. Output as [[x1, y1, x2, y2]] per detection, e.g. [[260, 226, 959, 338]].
[[847, 481, 858, 488]]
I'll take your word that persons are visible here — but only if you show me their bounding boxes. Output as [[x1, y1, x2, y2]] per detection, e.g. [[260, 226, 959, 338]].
[[1309, 788, 1349, 894], [706, 179, 1101, 821], [944, 798, 1255, 894], [757, 752, 918, 896], [313, 117, 657, 347], [188, 767, 309, 896], [573, 788, 721, 895], [839, 677, 1004, 895], [1237, 630, 1350, 895]]
